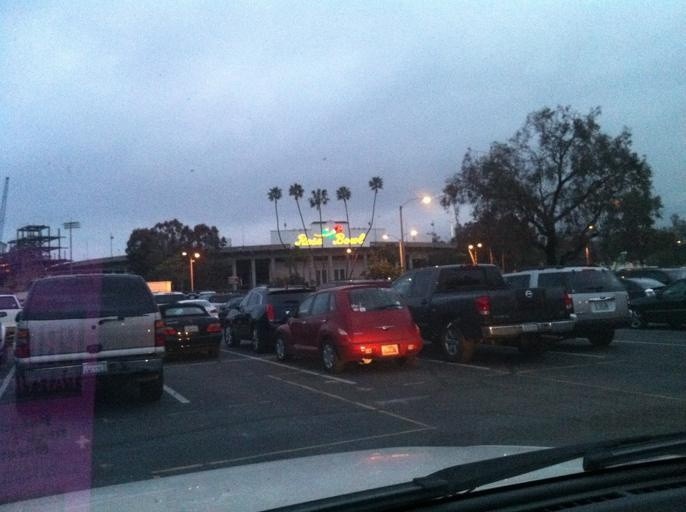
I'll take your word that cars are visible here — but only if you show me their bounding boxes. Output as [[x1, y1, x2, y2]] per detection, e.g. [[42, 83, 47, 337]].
[[150, 282, 316, 361], [618, 267, 686, 331], [273, 280, 423, 372], [0, 429, 685, 512]]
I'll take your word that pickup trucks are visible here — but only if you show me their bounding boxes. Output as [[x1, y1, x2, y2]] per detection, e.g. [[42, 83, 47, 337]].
[[389, 264, 577, 360]]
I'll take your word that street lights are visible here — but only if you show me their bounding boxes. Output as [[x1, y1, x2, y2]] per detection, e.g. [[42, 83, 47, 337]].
[[381, 229, 418, 272], [583, 224, 594, 267], [397, 197, 430, 270], [180, 250, 200, 292], [467, 243, 482, 264]]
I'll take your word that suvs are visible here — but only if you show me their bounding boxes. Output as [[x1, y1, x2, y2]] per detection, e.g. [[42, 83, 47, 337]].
[[504, 264, 632, 344], [12, 272, 163, 406], [0, 294, 23, 336]]
